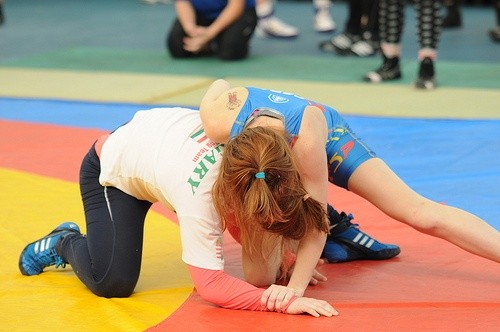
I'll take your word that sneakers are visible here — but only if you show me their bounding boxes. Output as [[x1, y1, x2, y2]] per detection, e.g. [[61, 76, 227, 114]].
[[19, 222, 80, 276], [255, 20, 299, 38], [417, 57, 435, 90], [319, 34, 375, 56], [324, 225, 401, 264], [364, 55, 401, 82], [314, 14, 335, 32]]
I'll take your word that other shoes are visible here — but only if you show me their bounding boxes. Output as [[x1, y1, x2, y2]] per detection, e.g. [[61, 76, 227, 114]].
[[489, 27, 500, 42], [440, 6, 460, 27]]
